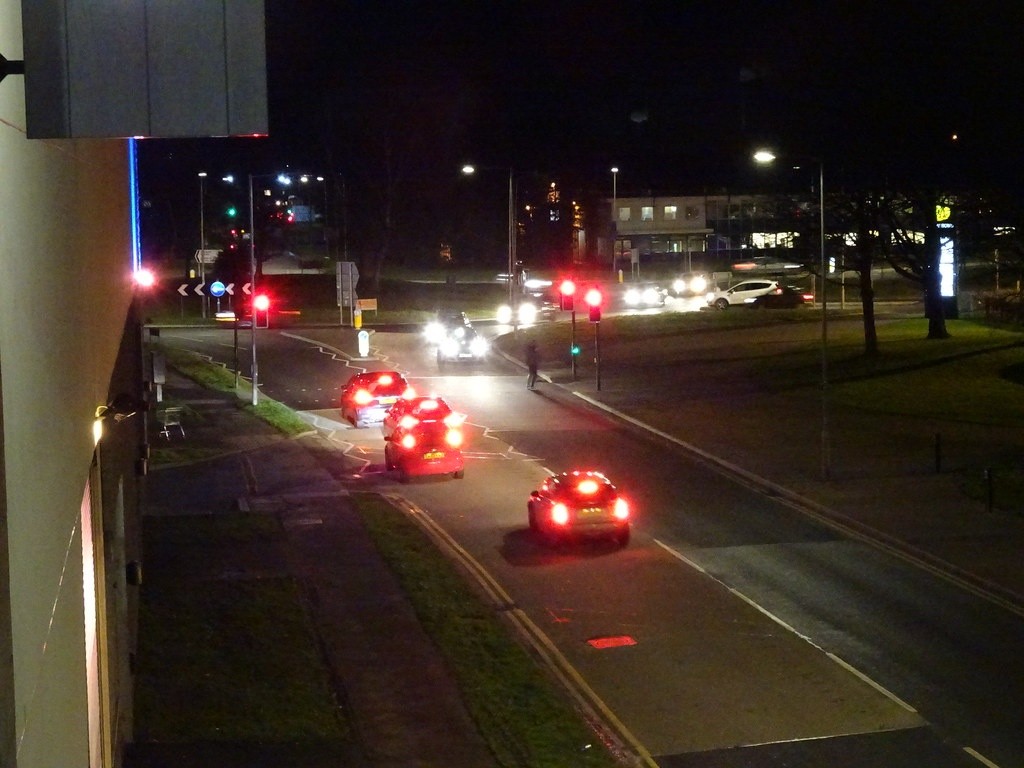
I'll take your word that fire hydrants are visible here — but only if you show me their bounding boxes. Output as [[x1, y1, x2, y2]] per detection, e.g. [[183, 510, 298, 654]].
[[617, 269, 623, 284]]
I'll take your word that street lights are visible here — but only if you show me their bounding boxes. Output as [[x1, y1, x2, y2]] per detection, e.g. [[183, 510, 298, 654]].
[[754, 151, 832, 480], [248, 173, 286, 324], [198, 172, 208, 318], [463, 164, 519, 344], [299, 177, 313, 247], [318, 176, 328, 229], [611, 166, 618, 274]]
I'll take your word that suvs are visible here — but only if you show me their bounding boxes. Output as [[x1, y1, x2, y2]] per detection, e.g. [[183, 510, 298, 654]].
[[427, 311, 473, 347], [526, 472, 632, 547], [708, 278, 782, 309]]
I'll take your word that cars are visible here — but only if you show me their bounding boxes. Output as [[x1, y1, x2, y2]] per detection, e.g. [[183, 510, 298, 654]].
[[227, 223, 253, 254], [676, 271, 716, 296], [504, 295, 557, 324], [626, 282, 668, 308], [383, 420, 464, 483], [733, 256, 801, 276], [384, 396, 454, 436], [342, 372, 418, 428], [436, 325, 487, 365]]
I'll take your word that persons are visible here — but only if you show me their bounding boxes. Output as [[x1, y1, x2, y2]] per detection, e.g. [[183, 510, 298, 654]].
[[525, 341, 538, 389]]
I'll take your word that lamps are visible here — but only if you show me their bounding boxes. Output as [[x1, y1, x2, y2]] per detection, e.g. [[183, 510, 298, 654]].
[[93, 395, 135, 422], [145, 318, 159, 337]]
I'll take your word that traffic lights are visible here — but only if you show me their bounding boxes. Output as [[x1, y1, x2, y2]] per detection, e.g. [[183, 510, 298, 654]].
[[586, 287, 603, 324], [560, 280, 576, 313], [253, 295, 269, 329], [573, 347, 580, 356]]
